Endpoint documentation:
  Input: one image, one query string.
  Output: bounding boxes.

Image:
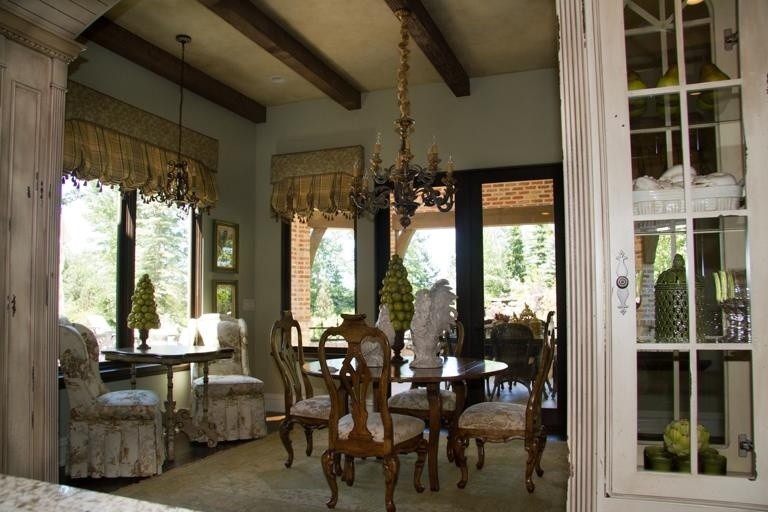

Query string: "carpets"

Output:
[111,420,569,510]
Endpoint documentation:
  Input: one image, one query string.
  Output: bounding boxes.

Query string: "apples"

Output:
[379,254,415,330]
[128,274,161,329]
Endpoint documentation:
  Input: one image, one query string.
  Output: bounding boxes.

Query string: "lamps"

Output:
[348,8,458,226]
[139,35,199,209]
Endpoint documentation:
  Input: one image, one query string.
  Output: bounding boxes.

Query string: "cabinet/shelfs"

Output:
[600,0,768,512]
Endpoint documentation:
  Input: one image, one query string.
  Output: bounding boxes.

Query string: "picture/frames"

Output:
[213,279,238,319]
[213,219,239,274]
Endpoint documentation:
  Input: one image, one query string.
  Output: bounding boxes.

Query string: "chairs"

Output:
[57,316,164,478]
[453,311,555,491]
[387,320,465,460]
[269,310,366,469]
[489,323,547,401]
[532,310,555,392]
[188,313,267,442]
[318,314,428,512]
